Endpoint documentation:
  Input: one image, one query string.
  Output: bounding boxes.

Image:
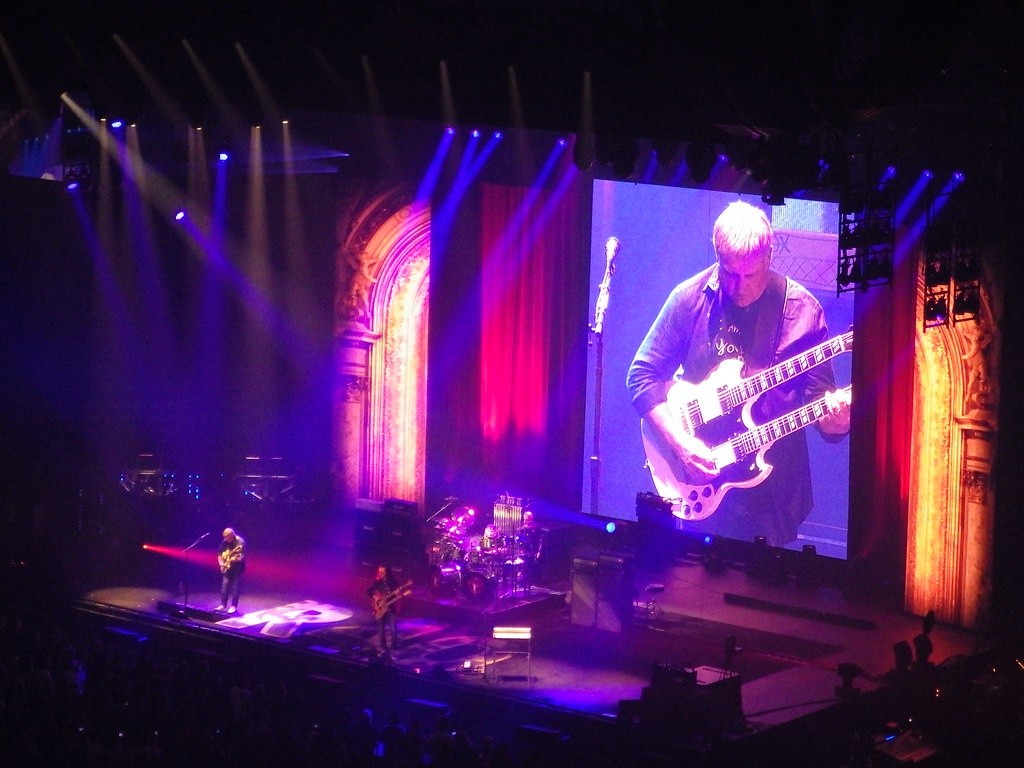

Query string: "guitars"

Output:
[641,325,853,522]
[373,579,413,620]
[220,545,242,573]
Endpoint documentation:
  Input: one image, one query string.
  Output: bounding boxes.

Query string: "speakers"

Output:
[572,551,635,634]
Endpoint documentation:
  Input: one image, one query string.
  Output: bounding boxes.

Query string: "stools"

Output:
[644,583,665,616]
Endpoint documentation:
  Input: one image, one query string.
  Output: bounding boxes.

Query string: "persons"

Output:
[623,202,848,546]
[517,511,541,582]
[374,563,400,664]
[217,528,248,610]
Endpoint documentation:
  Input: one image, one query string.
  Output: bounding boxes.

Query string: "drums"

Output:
[460,567,496,601]
[429,562,461,598]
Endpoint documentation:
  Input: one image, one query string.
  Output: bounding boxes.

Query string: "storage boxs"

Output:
[356,498,385,513]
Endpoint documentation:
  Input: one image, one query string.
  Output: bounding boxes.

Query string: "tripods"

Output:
[420,505,449,597]
[501,508,523,601]
[167,541,200,620]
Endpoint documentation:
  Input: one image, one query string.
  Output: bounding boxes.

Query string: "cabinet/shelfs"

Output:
[688,665,743,713]
[382,498,418,552]
[491,626,532,690]
[354,508,385,554]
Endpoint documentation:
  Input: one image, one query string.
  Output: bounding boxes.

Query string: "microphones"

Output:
[593,236,621,334]
[201,532,210,540]
